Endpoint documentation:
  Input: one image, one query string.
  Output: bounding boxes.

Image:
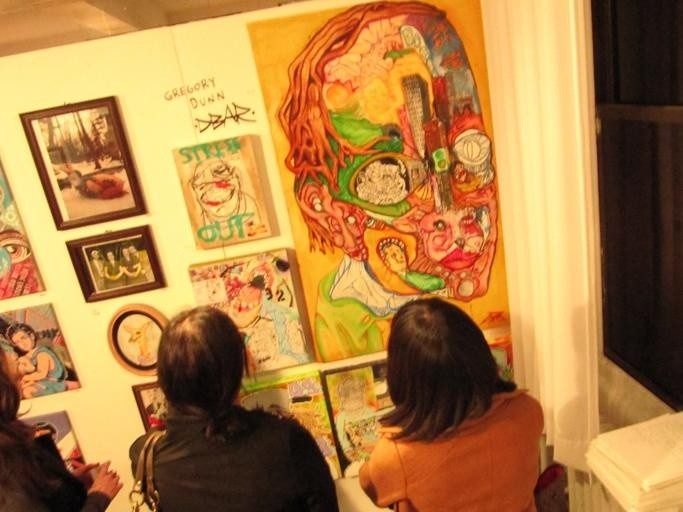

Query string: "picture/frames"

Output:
[66,225,167,304]
[107,303,172,376]
[132,381,169,436]
[18,95,147,231]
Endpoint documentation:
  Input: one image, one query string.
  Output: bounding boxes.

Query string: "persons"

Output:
[5,323,68,399]
[1,353,123,512]
[359,299,544,512]
[128,306,337,511]
